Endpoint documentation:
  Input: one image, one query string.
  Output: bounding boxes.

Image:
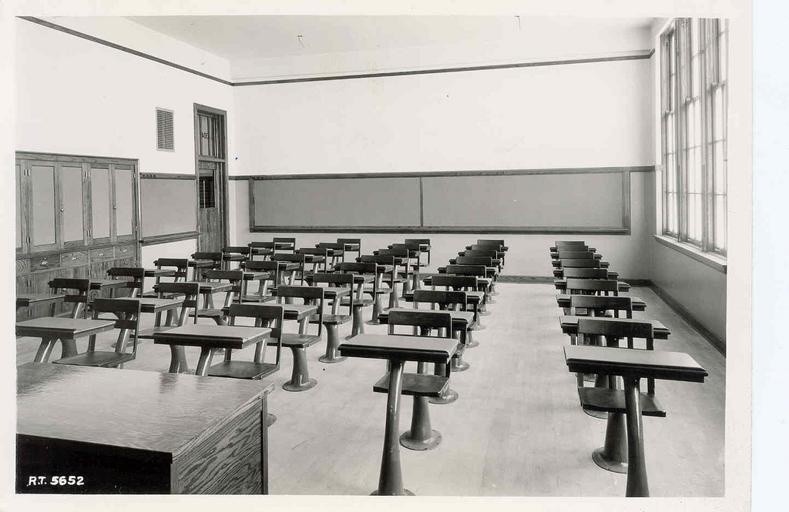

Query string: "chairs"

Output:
[16,238,708,497]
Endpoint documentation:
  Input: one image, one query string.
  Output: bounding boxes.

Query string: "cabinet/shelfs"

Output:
[15,151,142,335]
